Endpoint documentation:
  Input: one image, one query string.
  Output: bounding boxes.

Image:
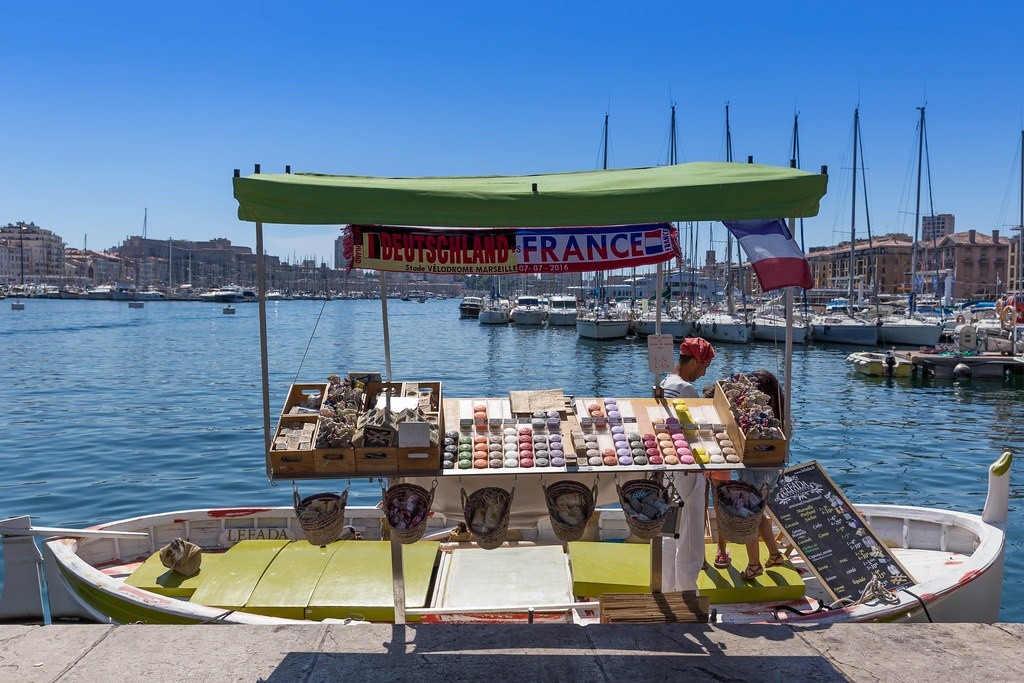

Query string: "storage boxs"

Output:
[713,377,787,463]
[269,381,442,475]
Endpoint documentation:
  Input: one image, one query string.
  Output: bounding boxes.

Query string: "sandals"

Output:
[744,562,763,582]
[714,550,730,566]
[765,552,784,566]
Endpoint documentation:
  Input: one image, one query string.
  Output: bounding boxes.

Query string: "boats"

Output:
[847,352,913,377]
[42,450,1016,625]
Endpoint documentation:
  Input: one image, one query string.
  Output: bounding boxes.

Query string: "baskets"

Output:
[294,491,348,546]
[382,483,433,544]
[616,479,674,539]
[711,480,770,544]
[461,487,515,550]
[542,480,598,542]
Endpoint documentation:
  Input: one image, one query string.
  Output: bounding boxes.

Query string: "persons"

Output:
[700,384,730,569]
[740,370,785,580]
[661,337,714,600]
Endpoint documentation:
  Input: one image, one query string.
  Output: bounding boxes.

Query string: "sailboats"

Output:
[0,254,456,304]
[457,99,1024,353]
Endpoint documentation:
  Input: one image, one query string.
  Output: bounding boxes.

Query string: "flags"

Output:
[722,219,814,291]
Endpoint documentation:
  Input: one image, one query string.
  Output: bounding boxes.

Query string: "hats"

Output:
[679,337,715,362]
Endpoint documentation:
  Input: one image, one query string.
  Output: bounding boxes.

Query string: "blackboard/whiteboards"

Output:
[763,459,919,608]
